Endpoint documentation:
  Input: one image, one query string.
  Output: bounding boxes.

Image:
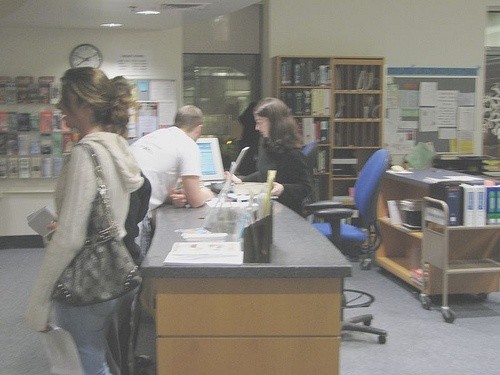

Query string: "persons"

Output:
[226,97,314,218]
[131,104,213,365]
[26,68,144,375]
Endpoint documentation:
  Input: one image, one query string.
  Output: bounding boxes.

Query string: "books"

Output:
[281,61,330,200]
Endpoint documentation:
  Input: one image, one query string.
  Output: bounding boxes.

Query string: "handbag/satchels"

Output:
[52,142,142,305]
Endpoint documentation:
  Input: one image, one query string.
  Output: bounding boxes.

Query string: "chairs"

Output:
[305,147,394,343]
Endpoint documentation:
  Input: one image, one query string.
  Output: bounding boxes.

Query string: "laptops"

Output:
[193,137,225,187]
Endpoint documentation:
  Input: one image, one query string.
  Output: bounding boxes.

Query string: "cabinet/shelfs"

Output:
[375,164,488,295]
[422,197,500,304]
[331,55,387,202]
[273,53,332,202]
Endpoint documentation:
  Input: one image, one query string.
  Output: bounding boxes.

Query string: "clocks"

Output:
[68,43,105,71]
